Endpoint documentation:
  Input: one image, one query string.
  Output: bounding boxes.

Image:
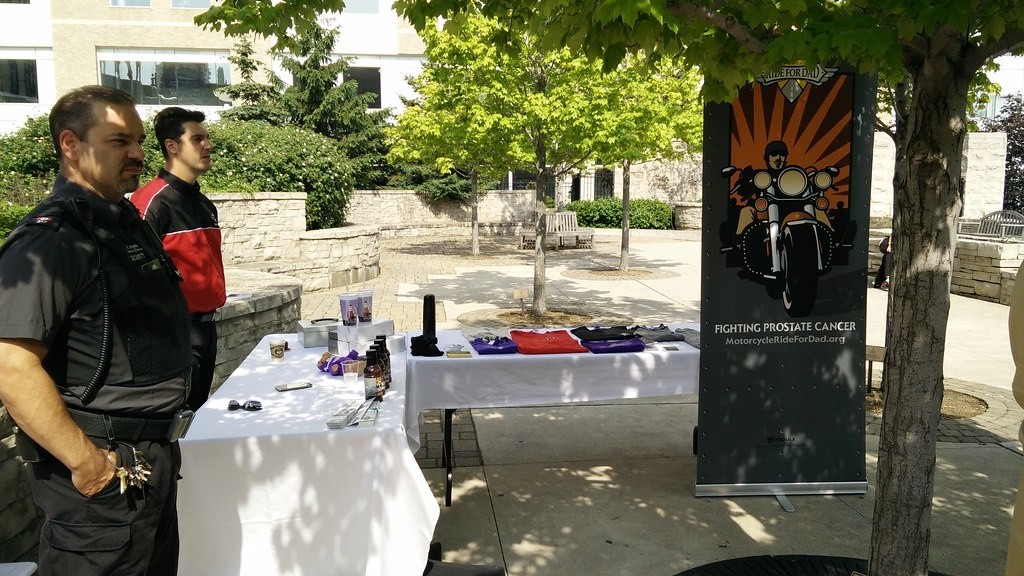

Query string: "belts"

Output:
[190,312,215,323]
[68,410,191,443]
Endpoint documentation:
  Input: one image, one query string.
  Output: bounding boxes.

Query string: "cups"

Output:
[339,293,357,325]
[269,341,286,363]
[355,291,372,322]
[341,360,359,385]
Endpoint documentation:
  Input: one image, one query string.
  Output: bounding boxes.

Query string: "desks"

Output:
[177,333,440,576]
[405,325,700,507]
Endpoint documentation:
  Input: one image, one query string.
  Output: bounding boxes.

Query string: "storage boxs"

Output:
[349,335,407,356]
[338,320,395,343]
[297,319,342,348]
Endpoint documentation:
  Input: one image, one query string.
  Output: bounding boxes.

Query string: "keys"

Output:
[116,450,151,494]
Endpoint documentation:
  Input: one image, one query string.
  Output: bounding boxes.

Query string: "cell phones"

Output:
[275,382,312,392]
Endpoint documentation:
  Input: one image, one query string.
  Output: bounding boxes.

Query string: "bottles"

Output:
[365,334,392,402]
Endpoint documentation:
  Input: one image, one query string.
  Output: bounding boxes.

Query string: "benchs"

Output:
[519,213,559,251]
[958,210,1024,244]
[557,212,595,249]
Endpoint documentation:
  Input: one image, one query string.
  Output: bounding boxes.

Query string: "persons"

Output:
[0,83,196,576]
[128,106,227,413]
